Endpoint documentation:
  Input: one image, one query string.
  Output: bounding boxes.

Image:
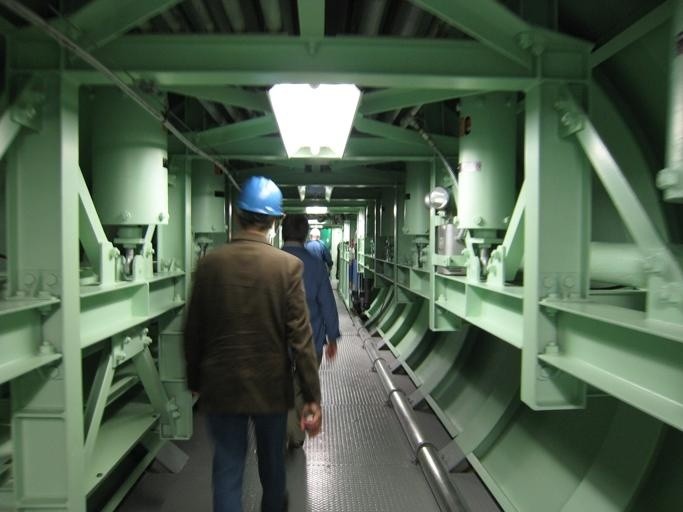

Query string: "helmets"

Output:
[236,176,284,218]
[309,228,320,237]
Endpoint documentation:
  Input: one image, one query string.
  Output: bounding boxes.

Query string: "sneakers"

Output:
[281,437,304,454]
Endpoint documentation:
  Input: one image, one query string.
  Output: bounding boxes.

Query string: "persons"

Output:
[179,173,323,511]
[279,212,342,448]
[306,228,333,277]
